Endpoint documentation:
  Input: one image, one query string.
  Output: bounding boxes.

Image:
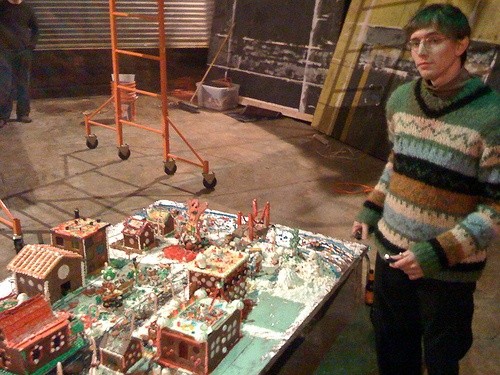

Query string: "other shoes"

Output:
[0,116,7,127]
[17,115,33,122]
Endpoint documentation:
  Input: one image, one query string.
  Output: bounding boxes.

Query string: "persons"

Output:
[351,4,500,375]
[0,0,39,129]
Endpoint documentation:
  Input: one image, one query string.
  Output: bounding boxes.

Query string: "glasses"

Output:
[407,35,450,47]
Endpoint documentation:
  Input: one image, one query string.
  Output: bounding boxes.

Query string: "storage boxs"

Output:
[196,80,241,111]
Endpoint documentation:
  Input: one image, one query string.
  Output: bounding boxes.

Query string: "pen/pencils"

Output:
[385,254,394,263]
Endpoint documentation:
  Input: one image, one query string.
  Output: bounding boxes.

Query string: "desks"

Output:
[0,199,368,375]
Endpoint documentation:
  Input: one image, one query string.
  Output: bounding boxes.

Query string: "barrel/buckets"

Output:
[110,73,138,122]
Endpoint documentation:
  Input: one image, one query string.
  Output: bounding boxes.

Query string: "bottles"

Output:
[364,269,375,305]
